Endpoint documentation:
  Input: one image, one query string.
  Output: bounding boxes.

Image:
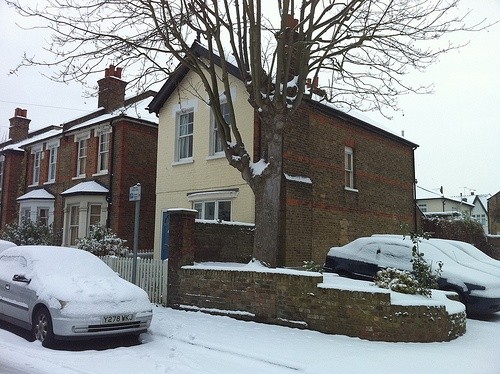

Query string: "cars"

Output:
[326,236,499,318]
[0,246,153,348]
[1,239,15,253]
